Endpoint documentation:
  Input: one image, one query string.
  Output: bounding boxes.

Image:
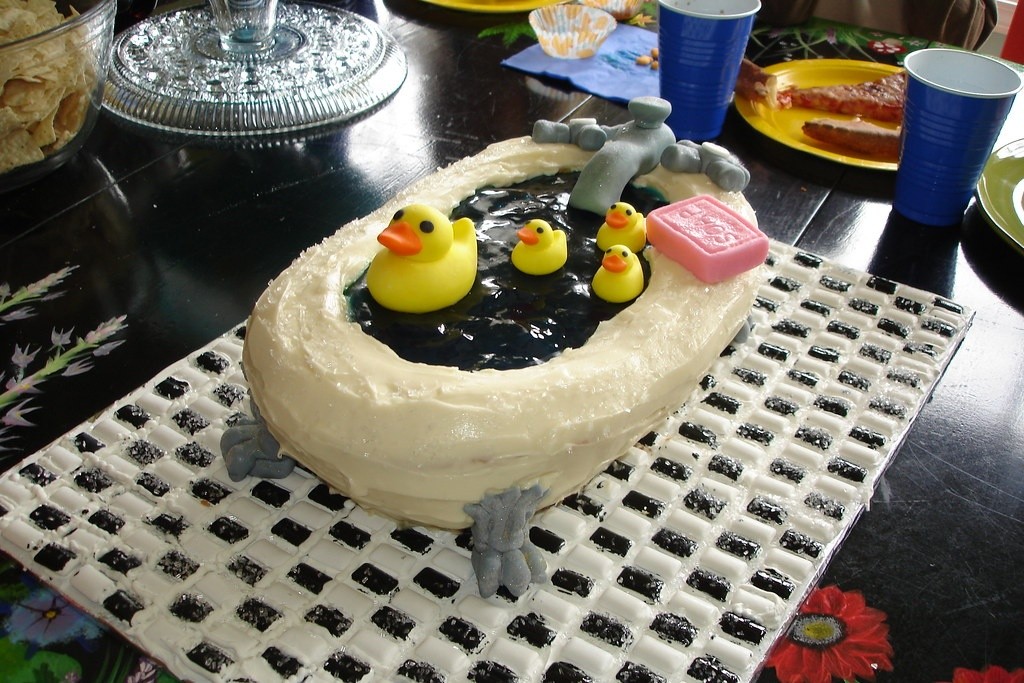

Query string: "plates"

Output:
[422,0,573,13]
[734,58,906,172]
[975,138,1024,255]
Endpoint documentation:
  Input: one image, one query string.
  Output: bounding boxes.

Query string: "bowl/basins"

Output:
[0,0,118,193]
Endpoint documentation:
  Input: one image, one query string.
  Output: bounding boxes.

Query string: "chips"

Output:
[0,0,104,172]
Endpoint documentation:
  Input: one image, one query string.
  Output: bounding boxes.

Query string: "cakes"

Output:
[216,95,769,597]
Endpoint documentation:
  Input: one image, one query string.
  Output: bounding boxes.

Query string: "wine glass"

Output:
[100,0,408,150]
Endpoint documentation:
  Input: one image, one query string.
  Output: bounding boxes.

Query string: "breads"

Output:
[780,70,906,120]
[801,117,900,156]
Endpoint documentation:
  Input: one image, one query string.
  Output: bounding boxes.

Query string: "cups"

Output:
[891,48,1024,226]
[658,0,761,142]
